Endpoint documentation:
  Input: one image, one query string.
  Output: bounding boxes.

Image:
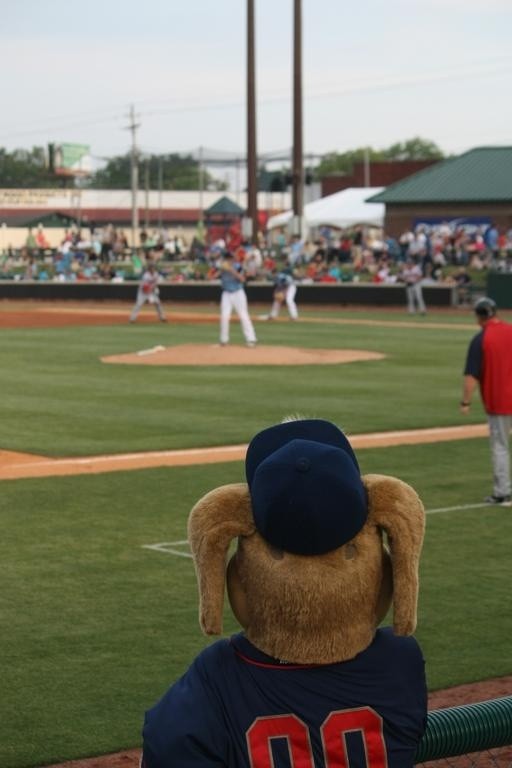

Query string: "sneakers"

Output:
[484,495,511,507]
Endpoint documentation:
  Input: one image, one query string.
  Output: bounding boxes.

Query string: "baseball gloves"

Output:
[275,291,285,302]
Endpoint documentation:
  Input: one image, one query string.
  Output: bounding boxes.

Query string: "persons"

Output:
[401,259,427,316]
[266,270,298,320]
[460,297,512,507]
[213,252,257,349]
[129,264,168,324]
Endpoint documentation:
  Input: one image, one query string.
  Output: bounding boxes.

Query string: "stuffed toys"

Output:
[140,414,428,768]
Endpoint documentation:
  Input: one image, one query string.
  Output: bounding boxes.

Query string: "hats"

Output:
[475,297,496,319]
[246,420,368,556]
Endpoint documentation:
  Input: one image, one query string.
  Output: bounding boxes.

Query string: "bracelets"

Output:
[461,401,471,406]
